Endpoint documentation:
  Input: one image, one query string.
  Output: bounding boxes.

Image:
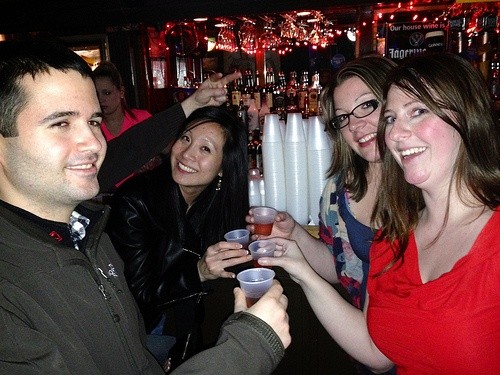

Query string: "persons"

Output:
[246,53,398,310]
[0,40,291,374]
[92,61,153,142]
[97,71,242,197]
[110,106,254,341]
[257,46,500,374]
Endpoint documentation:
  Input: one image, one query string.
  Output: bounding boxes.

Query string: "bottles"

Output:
[184,68,323,174]
[479,32,500,101]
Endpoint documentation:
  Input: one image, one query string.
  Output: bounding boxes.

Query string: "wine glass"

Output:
[214,10,328,54]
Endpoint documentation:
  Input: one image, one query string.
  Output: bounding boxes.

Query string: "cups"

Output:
[248,240,276,269]
[224,229,250,251]
[248,113,335,225]
[252,206,277,236]
[237,267,275,308]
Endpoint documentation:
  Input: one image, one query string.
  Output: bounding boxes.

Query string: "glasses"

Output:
[327,99,380,130]
